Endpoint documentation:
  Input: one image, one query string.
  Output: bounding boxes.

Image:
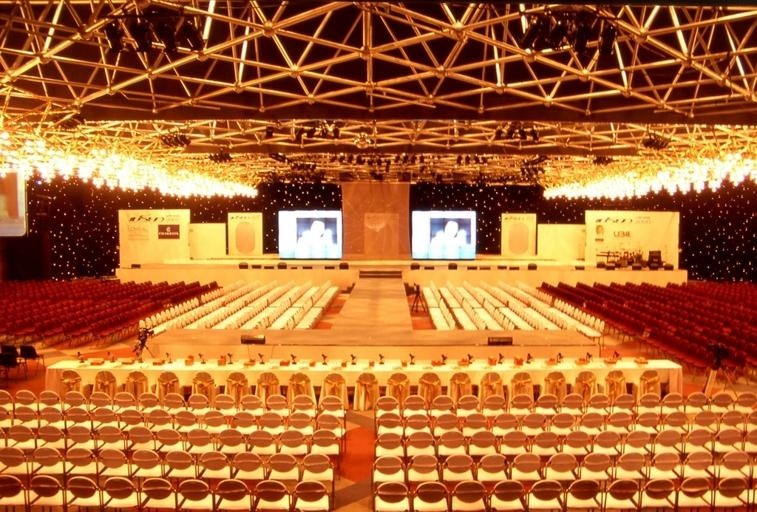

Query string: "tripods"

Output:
[132,343,155,358]
[412,295,426,313]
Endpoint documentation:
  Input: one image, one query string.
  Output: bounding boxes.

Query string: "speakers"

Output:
[487,336,513,345]
[649,250,662,268]
[240,334,265,344]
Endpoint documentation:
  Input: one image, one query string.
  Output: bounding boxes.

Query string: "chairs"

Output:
[0,279,757,512]
